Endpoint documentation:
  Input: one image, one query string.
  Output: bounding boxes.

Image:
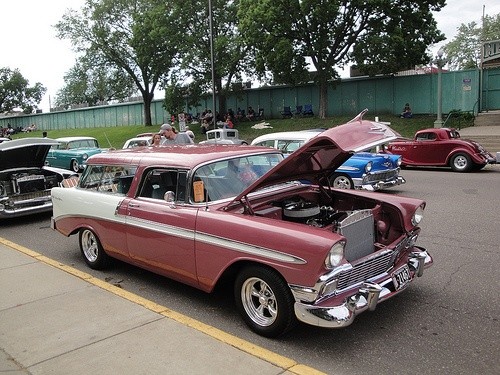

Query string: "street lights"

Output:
[433,49,447,128]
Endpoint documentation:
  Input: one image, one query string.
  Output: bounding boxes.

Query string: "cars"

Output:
[197,129,250,147]
[0,139,80,222]
[378,127,497,172]
[45,136,116,172]
[120,131,196,177]
[50,108,435,337]
[243,128,406,192]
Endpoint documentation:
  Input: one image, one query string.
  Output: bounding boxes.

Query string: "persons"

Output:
[22,122,36,133]
[151,133,162,146]
[201,119,209,133]
[234,107,243,121]
[4,127,17,133]
[400,102,411,118]
[185,107,236,128]
[158,123,195,143]
[178,113,186,131]
[166,111,175,125]
[245,105,256,121]
[184,126,195,141]
[43,131,48,138]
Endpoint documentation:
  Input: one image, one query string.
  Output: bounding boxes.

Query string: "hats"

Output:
[158,124,171,134]
